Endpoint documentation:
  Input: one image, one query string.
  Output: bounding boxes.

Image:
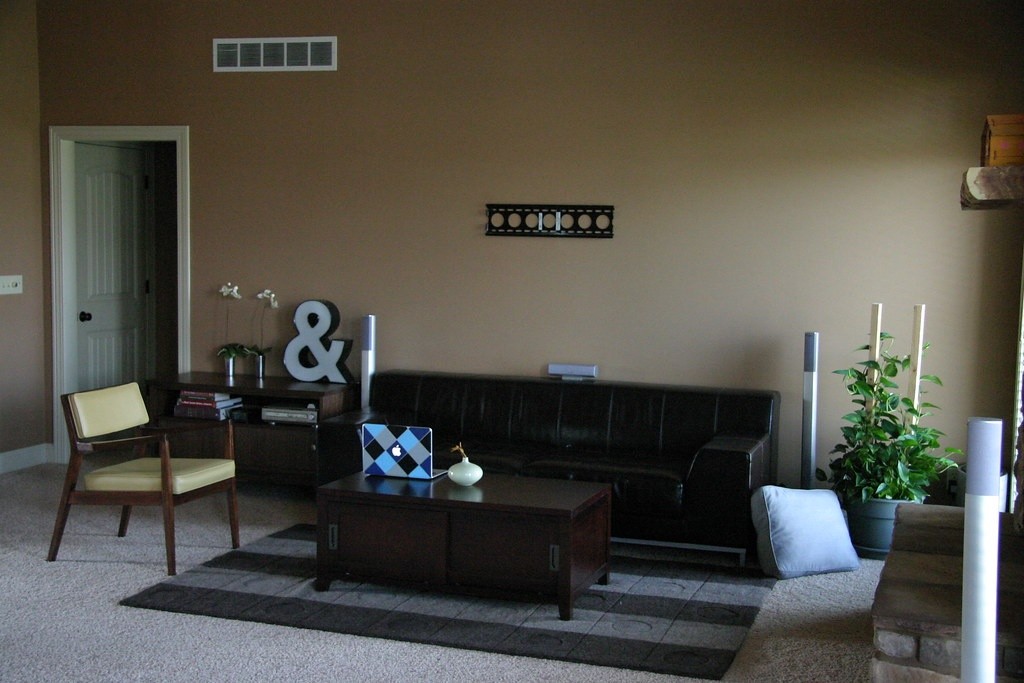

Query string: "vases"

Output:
[223,357,234,376]
[253,355,265,378]
[448,457,483,486]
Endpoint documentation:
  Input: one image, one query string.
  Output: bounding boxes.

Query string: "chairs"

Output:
[47,381,240,575]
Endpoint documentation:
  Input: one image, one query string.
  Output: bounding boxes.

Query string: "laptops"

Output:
[362,423,448,479]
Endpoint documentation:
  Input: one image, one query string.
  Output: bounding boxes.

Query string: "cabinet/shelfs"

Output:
[146,372,360,492]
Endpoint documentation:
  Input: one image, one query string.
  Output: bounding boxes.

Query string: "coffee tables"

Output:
[316,471,613,621]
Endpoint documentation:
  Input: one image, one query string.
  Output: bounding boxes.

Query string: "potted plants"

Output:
[815,332,964,561]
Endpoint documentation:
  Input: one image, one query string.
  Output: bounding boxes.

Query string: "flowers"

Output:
[216,282,246,359]
[249,289,279,356]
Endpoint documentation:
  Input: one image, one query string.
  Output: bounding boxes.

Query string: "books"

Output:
[174,391,242,421]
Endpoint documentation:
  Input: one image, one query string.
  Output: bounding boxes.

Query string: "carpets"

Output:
[116,524,778,683]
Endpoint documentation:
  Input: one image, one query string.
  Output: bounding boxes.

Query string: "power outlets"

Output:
[0,274,23,294]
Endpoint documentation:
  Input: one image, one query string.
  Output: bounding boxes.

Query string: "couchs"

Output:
[317,368,780,565]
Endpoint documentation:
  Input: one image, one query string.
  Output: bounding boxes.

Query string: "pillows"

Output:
[751,486,860,580]
